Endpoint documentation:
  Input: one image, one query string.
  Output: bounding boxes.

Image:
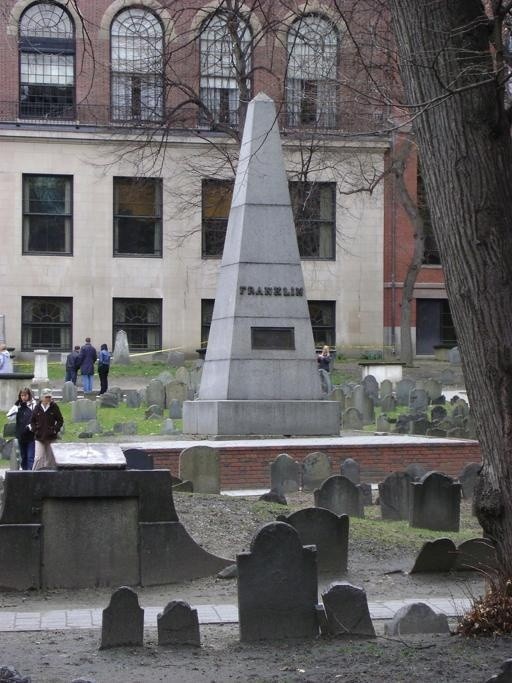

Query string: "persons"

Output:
[29,388,65,471]
[315,344,334,393]
[0,342,14,373]
[6,385,39,470]
[64,344,81,386]
[76,337,97,393]
[95,343,111,395]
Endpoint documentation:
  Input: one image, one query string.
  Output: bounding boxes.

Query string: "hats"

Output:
[40,387,53,398]
